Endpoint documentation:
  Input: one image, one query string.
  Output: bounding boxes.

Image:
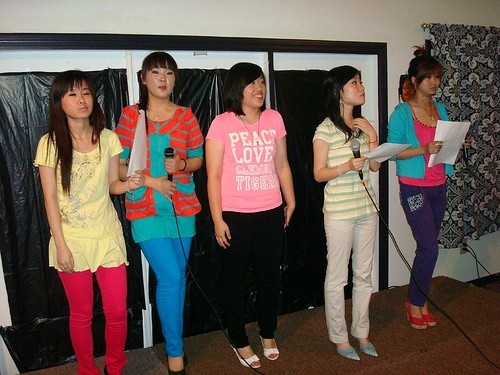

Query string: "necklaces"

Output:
[237,110,260,145]
[413,96,435,121]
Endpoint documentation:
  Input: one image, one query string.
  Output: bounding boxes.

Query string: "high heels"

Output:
[421,312,438,326]
[405,300,428,329]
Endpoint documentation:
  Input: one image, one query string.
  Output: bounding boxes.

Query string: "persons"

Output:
[385,44,454,329]
[312,65,381,361]
[34,68,146,375]
[114,51,204,375]
[206,62,296,369]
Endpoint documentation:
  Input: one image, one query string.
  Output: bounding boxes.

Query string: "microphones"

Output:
[165,148,174,183]
[349,139,363,180]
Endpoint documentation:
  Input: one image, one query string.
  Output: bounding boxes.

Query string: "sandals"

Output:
[258,334,280,360]
[230,343,261,369]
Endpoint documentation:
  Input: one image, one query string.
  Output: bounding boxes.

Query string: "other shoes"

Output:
[166,349,187,375]
[337,346,361,361]
[360,342,379,357]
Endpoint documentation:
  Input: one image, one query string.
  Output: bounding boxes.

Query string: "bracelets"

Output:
[126,181,136,194]
[337,164,345,176]
[368,137,377,145]
[181,158,187,171]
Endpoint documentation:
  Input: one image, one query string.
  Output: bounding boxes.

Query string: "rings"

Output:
[168,191,172,193]
[64,267,68,269]
